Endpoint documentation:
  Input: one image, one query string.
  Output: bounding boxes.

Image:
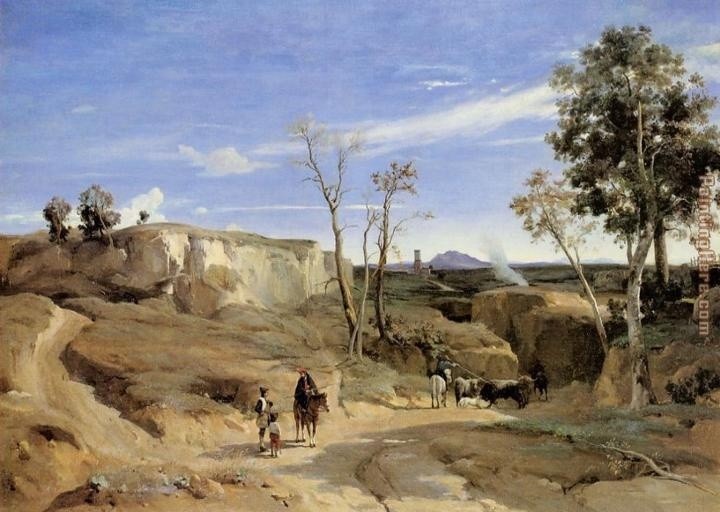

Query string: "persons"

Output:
[253,384,274,452]
[268,410,282,459]
[293,367,319,418]
[435,354,461,392]
[529,357,544,379]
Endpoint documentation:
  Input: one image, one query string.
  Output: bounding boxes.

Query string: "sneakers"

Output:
[259,446,269,452]
[269,454,281,458]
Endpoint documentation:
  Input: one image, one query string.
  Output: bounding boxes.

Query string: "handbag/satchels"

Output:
[256,412,269,429]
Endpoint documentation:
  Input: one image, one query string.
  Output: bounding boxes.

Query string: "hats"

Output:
[296,368,308,373]
[260,386,268,393]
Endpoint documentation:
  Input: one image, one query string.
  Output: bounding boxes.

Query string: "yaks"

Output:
[454,375,538,410]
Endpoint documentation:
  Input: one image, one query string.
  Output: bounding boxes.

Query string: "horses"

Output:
[292,392,330,448]
[429,367,451,409]
[527,365,549,401]
